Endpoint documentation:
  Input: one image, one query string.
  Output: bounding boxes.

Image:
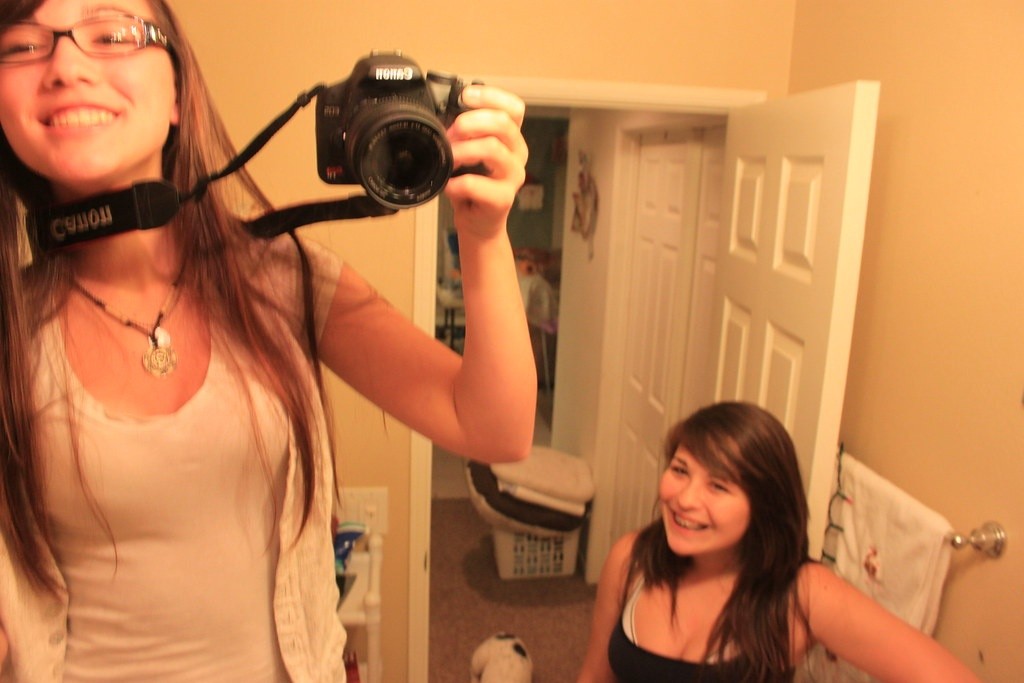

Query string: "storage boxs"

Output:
[491,522,580,580]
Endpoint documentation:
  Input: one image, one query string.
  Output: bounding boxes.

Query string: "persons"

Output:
[0,0,537,683]
[577,402,981,683]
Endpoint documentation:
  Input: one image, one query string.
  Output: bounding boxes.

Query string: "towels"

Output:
[487,442,595,517]
[823,451,951,660]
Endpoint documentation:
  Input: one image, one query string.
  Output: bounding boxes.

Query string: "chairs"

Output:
[436,227,466,353]
[330,485,389,683]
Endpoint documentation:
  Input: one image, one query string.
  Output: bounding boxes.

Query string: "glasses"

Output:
[0,15,174,64]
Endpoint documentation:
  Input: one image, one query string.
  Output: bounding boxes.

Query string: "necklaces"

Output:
[70,253,189,377]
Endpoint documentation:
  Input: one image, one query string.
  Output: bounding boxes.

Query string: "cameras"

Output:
[314,54,485,211]
